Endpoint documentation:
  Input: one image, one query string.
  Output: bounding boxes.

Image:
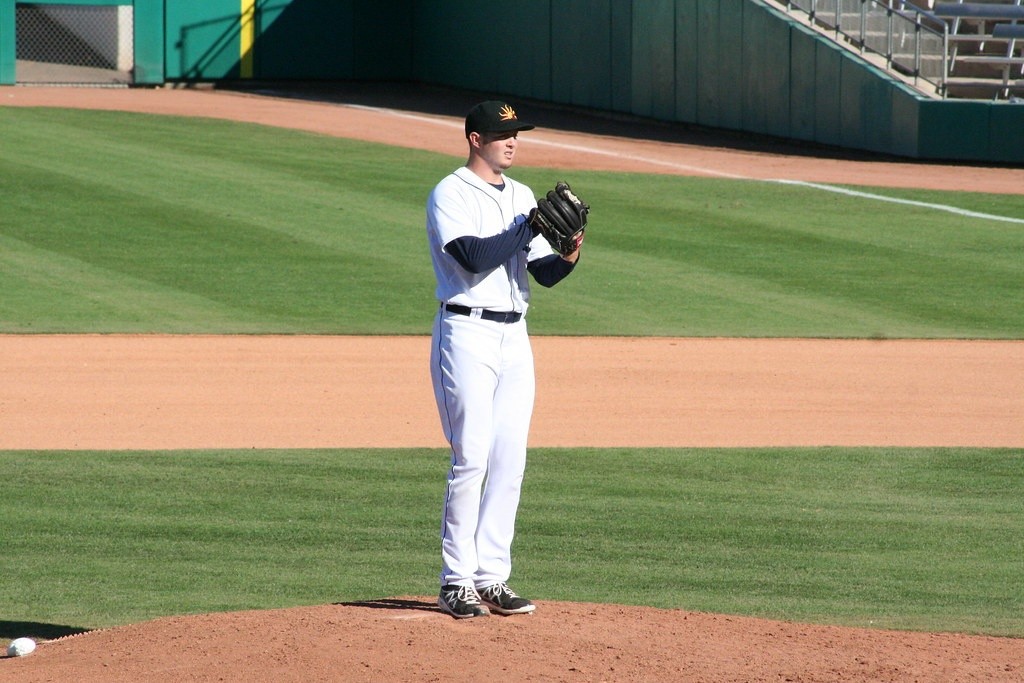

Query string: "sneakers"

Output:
[477,582,536,614]
[438,584,489,617]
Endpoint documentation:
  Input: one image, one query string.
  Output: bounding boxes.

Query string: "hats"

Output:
[465,101,535,132]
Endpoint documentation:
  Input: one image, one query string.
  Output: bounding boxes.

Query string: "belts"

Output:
[441,301,521,324]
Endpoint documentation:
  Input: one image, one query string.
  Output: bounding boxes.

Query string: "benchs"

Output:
[917,0,1024,91]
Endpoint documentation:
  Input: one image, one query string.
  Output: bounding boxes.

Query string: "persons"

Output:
[426,102,581,619]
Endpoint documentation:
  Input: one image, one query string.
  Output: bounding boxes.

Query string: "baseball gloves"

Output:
[529,178,591,257]
